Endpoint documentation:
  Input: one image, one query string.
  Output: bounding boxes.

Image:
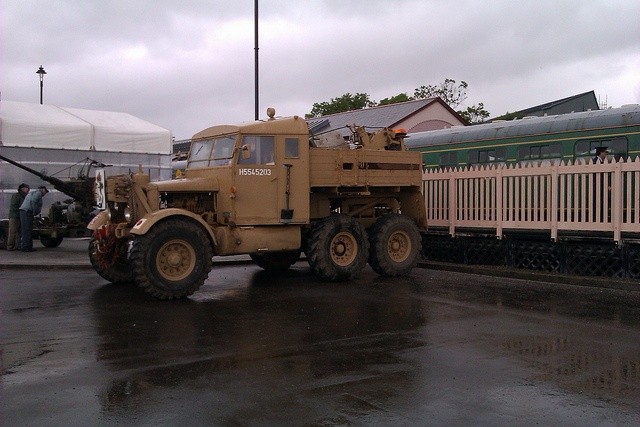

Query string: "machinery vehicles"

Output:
[0,155,113,248]
[89,108,427,299]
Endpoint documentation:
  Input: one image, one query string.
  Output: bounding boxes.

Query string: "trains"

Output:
[403,105,640,211]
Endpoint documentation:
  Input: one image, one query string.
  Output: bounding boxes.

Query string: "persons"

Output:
[6,182,30,250]
[592,144,608,221]
[17,184,50,250]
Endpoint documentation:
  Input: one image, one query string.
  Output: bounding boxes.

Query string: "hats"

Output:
[38,186,49,193]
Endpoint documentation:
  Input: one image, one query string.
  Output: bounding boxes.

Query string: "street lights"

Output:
[36,64,47,104]
